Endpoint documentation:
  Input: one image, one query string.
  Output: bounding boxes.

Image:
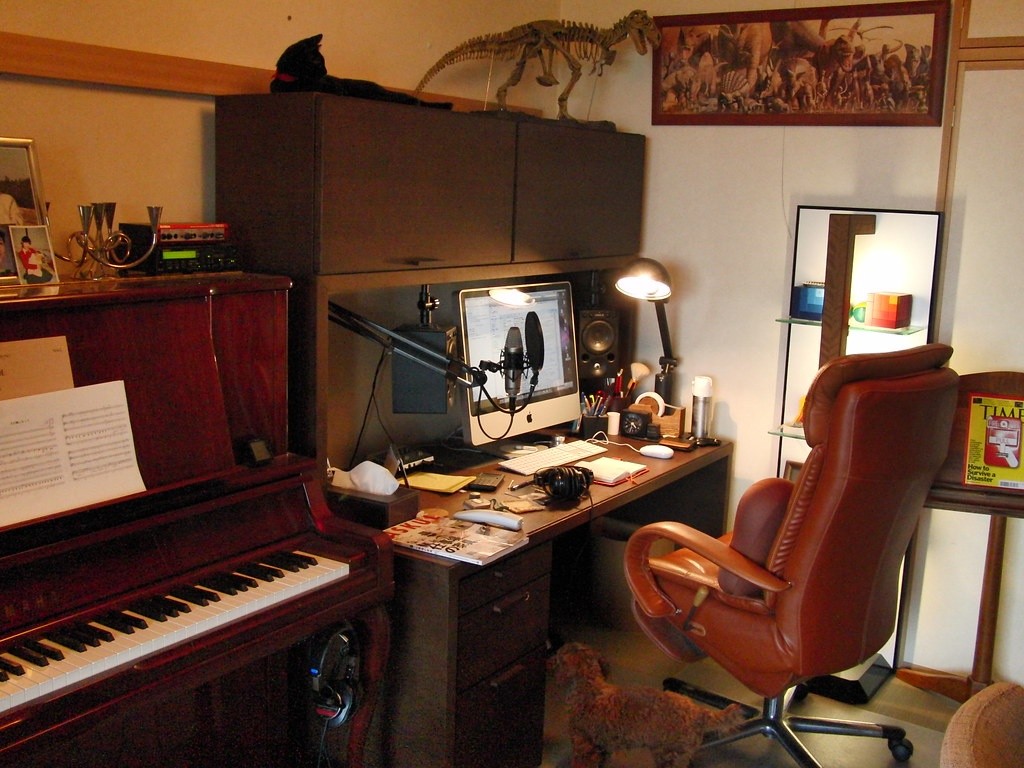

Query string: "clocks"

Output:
[620,410,652,438]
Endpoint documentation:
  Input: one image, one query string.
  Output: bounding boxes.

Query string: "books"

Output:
[574,457,649,486]
[383,515,530,566]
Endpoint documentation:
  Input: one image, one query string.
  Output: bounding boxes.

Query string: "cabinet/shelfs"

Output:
[214,91,647,280]
[767,313,926,440]
[386,428,734,768]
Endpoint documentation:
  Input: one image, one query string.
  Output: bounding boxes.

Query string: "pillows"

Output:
[717,479,796,597]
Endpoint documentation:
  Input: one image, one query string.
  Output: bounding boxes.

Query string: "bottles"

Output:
[691,376,713,439]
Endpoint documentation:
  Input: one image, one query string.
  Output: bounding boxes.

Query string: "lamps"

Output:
[613,258,679,415]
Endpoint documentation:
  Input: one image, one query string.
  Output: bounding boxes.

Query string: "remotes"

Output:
[454,509,523,531]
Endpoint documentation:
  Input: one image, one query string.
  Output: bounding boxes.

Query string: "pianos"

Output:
[0,268,397,768]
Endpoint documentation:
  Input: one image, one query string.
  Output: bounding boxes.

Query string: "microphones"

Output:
[504,327,524,415]
[525,310,545,395]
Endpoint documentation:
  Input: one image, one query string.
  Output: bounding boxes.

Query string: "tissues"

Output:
[324,460,419,529]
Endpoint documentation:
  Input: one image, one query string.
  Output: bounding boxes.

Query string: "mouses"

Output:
[640,444,674,459]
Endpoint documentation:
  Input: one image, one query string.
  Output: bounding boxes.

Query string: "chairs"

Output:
[938,681,1024,768]
[622,342,961,768]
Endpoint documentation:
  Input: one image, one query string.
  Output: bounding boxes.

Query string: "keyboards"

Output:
[497,440,609,476]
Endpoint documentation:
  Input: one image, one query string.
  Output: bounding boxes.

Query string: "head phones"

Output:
[309,622,365,730]
[534,466,594,505]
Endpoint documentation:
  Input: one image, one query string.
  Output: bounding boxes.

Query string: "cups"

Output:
[610,398,632,432]
[583,414,608,440]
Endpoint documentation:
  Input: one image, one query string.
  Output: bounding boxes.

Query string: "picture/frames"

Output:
[651,0,952,127]
[8,225,61,285]
[0,137,52,286]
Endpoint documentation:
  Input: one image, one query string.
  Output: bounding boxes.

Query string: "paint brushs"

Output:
[625,363,650,397]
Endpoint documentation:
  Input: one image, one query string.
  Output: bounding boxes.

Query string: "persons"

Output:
[0,230,15,276]
[18,236,53,284]
[0,193,24,225]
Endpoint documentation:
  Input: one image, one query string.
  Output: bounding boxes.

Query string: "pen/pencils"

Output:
[580,390,612,416]
[507,479,514,489]
[512,480,532,491]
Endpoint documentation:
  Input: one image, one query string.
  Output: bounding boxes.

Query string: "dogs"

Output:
[542,641,745,768]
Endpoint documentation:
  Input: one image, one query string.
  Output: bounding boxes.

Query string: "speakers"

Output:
[574,308,619,380]
[392,323,457,415]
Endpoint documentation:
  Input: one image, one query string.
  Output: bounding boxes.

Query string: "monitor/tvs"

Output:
[453,280,581,460]
